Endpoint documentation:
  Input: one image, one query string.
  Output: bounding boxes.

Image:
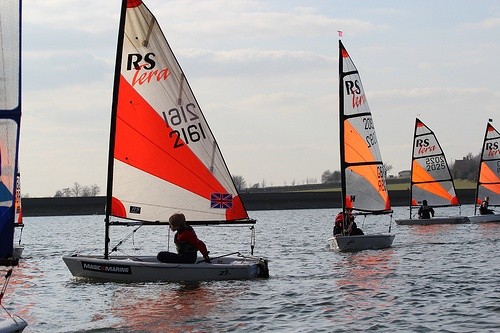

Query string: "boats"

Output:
[0,268,27,333]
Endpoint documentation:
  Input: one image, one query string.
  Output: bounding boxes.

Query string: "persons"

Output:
[479,196,495,215]
[418,200,435,219]
[333,207,364,236]
[156,214,212,264]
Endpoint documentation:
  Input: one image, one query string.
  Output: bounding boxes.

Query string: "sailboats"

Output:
[328,30,395,250]
[467,119,500,222]
[62,0,269,283]
[394,117,466,225]
[1,1,21,267]
[16,169,25,260]
